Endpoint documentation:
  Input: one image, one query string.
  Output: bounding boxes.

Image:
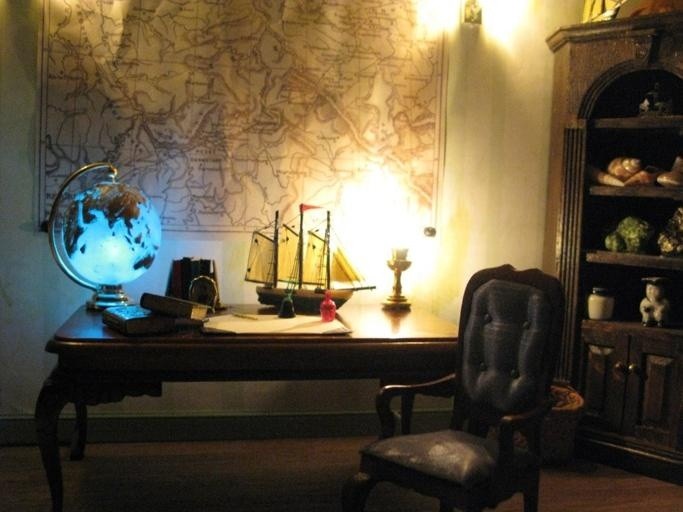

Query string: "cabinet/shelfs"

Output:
[545,322,682,487]
[546,0,683,329]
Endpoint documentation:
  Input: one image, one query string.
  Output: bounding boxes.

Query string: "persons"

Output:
[640,277,670,330]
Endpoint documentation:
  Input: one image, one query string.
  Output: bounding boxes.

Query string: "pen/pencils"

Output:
[233,313,258,321]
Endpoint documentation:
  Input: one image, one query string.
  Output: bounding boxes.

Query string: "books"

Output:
[102,293,211,339]
[166,257,222,312]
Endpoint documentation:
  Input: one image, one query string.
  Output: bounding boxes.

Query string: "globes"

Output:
[47,161,162,311]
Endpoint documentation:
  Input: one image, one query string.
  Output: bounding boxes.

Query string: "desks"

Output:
[34,292,460,511]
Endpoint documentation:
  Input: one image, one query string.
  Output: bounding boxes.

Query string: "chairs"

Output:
[343,262,567,511]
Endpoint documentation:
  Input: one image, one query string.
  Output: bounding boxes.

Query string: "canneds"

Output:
[586,286,614,320]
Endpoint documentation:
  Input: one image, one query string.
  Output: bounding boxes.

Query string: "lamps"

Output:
[381,249,413,314]
[460,0,483,29]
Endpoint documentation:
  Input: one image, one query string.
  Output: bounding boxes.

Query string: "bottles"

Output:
[319,290,336,322]
